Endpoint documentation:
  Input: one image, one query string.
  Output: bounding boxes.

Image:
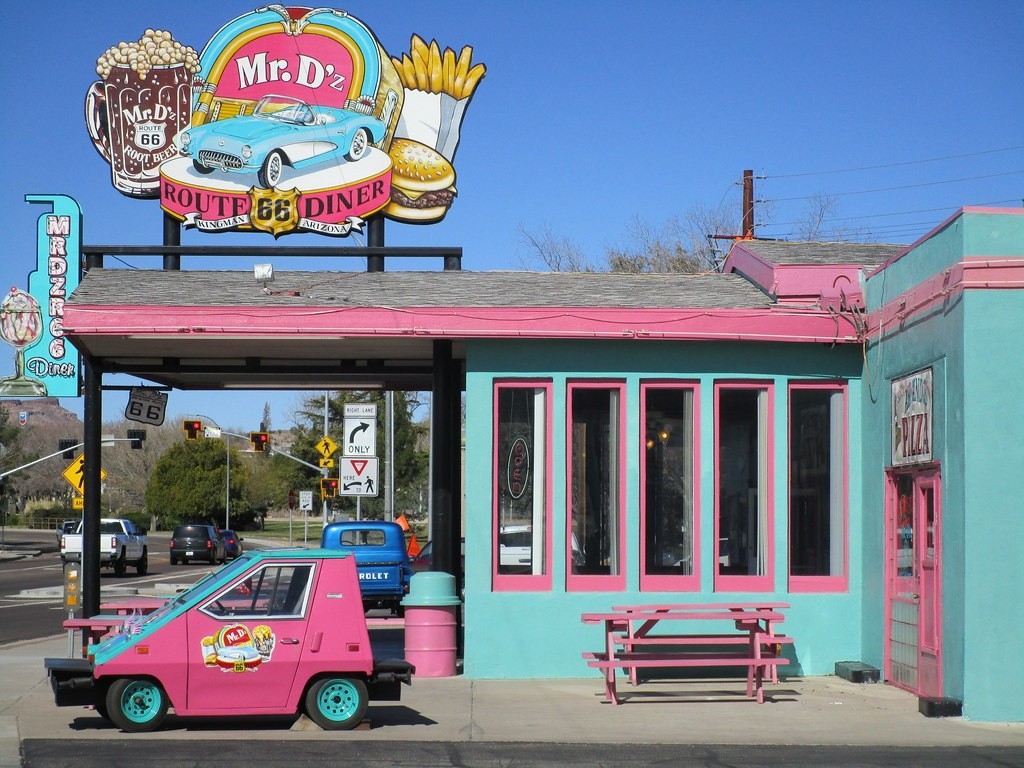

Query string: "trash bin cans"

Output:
[400,570,462,680]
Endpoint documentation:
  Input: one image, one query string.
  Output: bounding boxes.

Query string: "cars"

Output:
[56,520,78,548]
[218,529,244,560]
[43,545,415,730]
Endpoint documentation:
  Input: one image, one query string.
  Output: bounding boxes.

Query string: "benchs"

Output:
[581,634,794,666]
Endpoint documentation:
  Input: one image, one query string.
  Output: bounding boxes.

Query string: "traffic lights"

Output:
[183,420,202,441]
[250,431,270,452]
[320,478,338,498]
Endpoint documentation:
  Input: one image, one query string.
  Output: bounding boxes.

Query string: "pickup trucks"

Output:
[320,521,416,618]
[60,519,149,578]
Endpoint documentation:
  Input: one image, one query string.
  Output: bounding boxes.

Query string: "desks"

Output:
[581,613,787,705]
[612,602,791,686]
[62,599,274,638]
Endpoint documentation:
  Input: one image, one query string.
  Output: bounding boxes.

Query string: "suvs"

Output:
[170,524,228,566]
